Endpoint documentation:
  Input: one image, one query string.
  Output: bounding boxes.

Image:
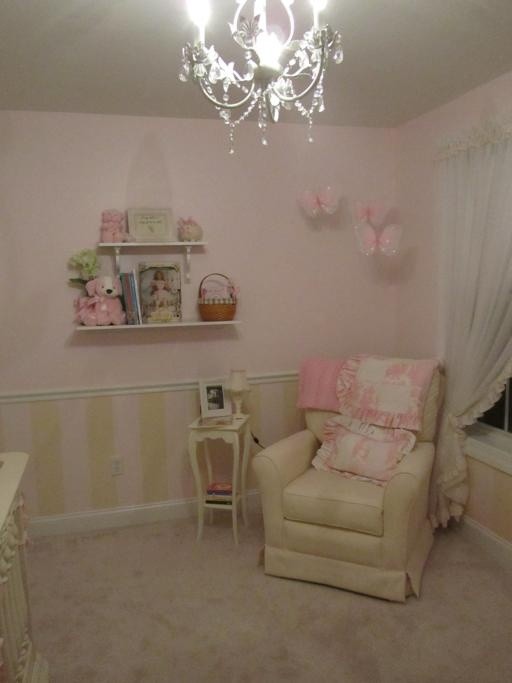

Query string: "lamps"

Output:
[225,371,251,419]
[178,0,346,158]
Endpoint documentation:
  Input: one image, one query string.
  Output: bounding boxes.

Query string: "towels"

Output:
[294,355,346,414]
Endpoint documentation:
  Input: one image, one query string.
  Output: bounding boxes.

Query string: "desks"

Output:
[189,413,252,548]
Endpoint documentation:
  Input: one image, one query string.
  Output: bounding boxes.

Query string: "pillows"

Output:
[310,416,419,485]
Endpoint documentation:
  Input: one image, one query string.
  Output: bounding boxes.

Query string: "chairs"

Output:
[255,354,447,603]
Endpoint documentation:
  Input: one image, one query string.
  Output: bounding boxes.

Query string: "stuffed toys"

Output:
[176,216,203,241]
[77,274,128,327]
[100,207,127,243]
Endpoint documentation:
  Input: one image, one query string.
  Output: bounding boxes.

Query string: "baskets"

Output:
[198,273,237,321]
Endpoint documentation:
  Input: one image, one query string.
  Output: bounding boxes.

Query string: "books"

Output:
[119,268,144,326]
[206,482,239,506]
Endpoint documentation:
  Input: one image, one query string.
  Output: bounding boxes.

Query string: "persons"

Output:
[144,269,175,313]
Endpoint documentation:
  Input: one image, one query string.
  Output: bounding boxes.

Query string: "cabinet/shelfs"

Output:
[71,242,243,332]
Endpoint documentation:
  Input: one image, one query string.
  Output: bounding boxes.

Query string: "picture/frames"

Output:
[199,376,231,420]
[127,208,174,240]
